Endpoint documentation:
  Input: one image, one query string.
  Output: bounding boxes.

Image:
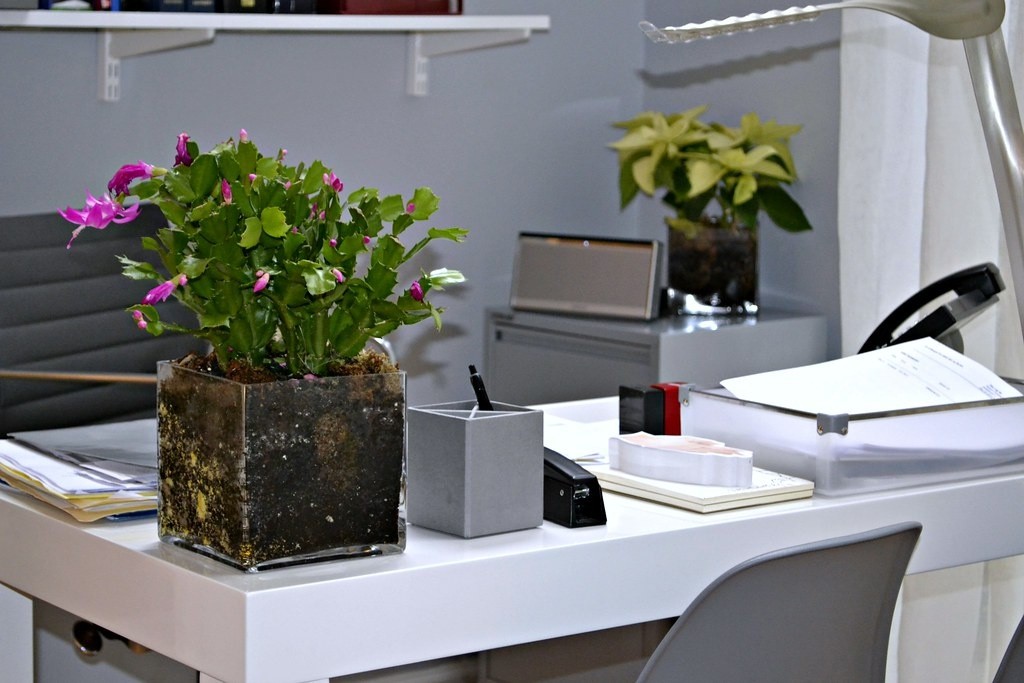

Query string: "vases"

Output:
[155,359,409,575]
[667,223,759,316]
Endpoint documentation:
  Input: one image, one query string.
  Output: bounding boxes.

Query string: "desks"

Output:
[0,397,1024,683]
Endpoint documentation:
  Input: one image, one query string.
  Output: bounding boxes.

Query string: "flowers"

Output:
[603,103,814,240]
[54,124,470,379]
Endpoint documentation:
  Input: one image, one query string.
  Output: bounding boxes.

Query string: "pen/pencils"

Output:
[466,364,494,410]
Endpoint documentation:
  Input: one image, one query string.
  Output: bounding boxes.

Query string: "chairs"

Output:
[635,521,923,683]
[0,203,213,438]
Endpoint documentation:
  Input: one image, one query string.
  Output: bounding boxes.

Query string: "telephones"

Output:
[855,261,1007,357]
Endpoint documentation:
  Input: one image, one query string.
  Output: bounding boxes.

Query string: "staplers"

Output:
[542,443,608,530]
